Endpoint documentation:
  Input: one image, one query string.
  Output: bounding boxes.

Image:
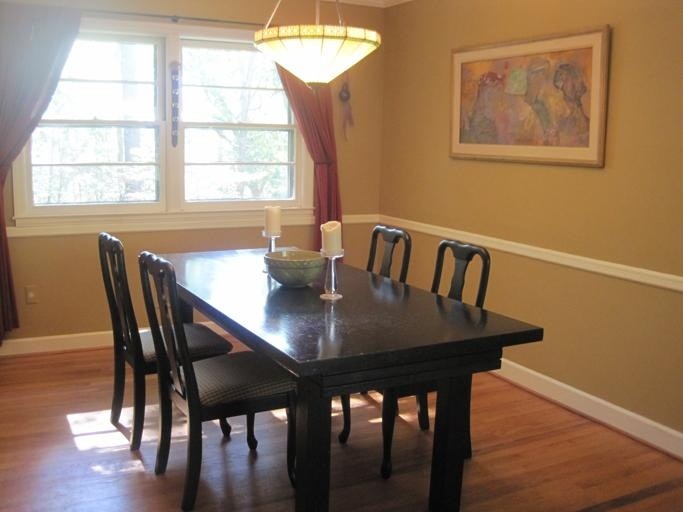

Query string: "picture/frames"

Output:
[448,23,612,168]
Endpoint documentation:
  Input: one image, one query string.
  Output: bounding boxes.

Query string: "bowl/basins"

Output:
[264,249,327,289]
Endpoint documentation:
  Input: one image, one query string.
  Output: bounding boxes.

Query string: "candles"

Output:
[321,219,343,256]
[263,204,282,234]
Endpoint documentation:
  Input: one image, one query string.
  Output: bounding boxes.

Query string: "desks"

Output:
[157,245,544,512]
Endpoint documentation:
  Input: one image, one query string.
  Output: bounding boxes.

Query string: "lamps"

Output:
[254,0,379,97]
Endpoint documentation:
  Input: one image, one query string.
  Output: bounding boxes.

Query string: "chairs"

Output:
[366,225,413,282]
[139,251,298,512]
[339,240,491,480]
[97,232,232,452]
